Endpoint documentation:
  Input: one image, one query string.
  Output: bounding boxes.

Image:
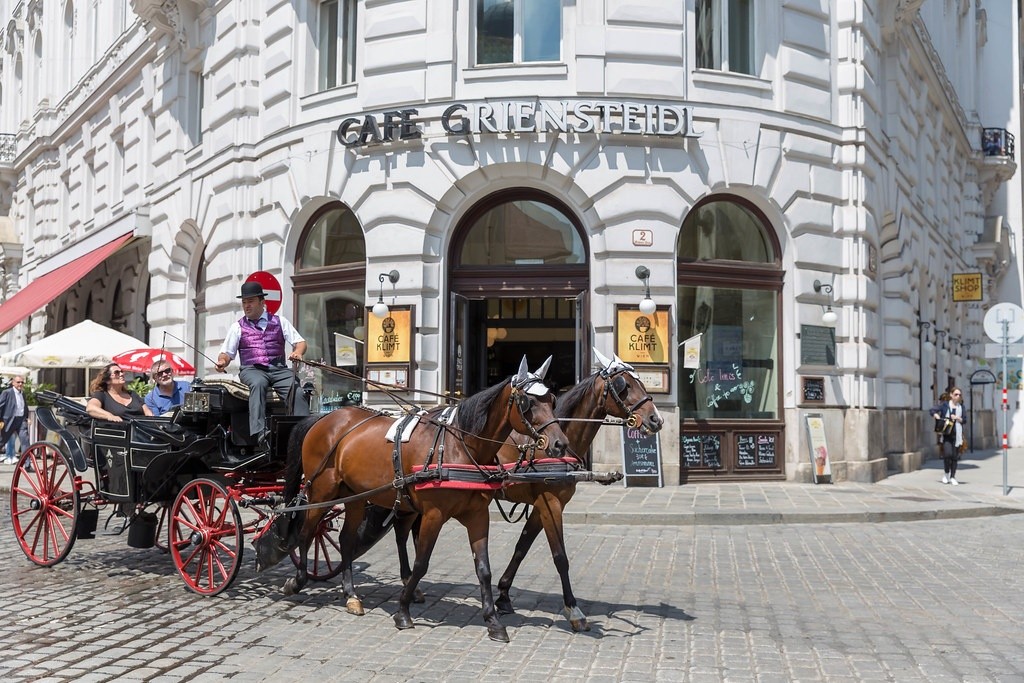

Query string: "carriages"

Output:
[9,345,666,643]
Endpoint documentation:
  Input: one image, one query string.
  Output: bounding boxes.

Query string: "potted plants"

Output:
[22,377,57,457]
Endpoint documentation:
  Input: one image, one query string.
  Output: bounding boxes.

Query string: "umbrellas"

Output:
[112,348,196,387]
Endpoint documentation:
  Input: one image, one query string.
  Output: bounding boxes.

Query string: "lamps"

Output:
[354,304,364,340]
[635,266,657,315]
[917,321,973,368]
[813,280,837,322]
[372,270,400,317]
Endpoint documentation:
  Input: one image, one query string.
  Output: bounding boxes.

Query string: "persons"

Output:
[86,362,154,422]
[0,377,37,473]
[144,360,191,416]
[215,283,310,445]
[4,431,20,465]
[930,388,967,486]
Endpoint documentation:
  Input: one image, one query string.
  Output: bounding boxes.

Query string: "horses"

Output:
[284,353,570,643]
[360,347,664,631]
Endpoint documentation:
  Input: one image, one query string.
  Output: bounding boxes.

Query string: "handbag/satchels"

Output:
[933,402,954,435]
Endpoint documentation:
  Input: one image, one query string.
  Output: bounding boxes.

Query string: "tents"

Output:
[2,320,152,397]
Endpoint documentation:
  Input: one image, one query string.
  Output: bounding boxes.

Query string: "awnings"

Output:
[0,232,133,333]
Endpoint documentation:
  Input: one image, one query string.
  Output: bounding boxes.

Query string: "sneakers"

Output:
[943,473,949,483]
[4,459,12,464]
[950,478,959,486]
[12,457,20,464]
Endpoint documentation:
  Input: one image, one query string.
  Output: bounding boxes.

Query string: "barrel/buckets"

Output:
[77,501,99,540]
[128,507,158,548]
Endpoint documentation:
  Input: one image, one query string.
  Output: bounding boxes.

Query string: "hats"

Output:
[236,283,268,298]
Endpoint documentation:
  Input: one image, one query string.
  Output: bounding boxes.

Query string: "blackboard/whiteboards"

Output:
[702,435,721,468]
[758,435,776,466]
[737,435,755,466]
[681,435,701,468]
[620,424,662,477]
[800,376,825,404]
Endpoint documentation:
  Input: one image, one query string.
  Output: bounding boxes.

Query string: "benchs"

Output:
[194,383,286,416]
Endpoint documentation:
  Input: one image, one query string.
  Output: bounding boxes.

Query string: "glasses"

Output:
[153,368,170,378]
[109,369,124,377]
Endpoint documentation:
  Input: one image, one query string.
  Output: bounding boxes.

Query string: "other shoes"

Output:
[26,466,36,472]
[256,430,270,444]
